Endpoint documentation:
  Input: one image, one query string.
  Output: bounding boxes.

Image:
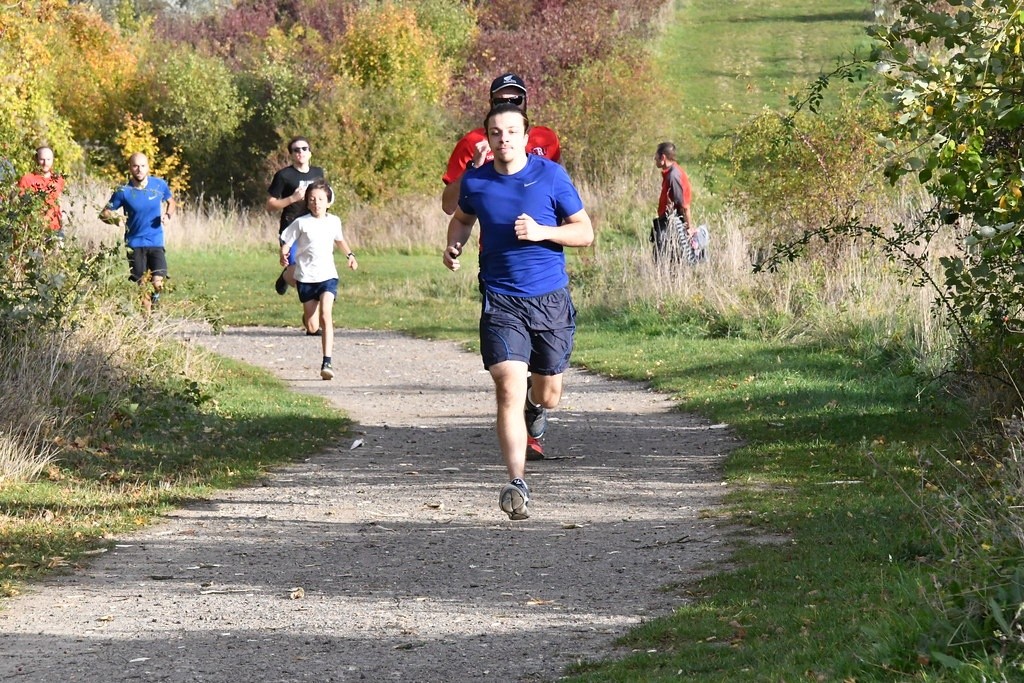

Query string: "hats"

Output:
[490,74,527,94]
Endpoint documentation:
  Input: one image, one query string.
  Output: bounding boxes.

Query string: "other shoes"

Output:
[306,329,322,335]
[151,291,159,306]
[276,266,289,294]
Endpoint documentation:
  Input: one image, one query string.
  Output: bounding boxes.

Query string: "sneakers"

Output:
[320,362,333,380]
[499,479,531,520]
[526,436,544,461]
[524,377,547,440]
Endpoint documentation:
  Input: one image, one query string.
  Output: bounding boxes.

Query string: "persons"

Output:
[98,151,174,331]
[441,105,595,518]
[453,74,565,459]
[651,143,691,266]
[278,180,357,380]
[15,147,67,266]
[265,136,335,335]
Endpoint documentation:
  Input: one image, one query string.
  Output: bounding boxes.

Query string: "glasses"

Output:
[294,147,308,152]
[491,95,525,105]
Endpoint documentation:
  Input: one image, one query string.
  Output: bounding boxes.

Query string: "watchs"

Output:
[165,212,171,220]
[347,253,355,258]
